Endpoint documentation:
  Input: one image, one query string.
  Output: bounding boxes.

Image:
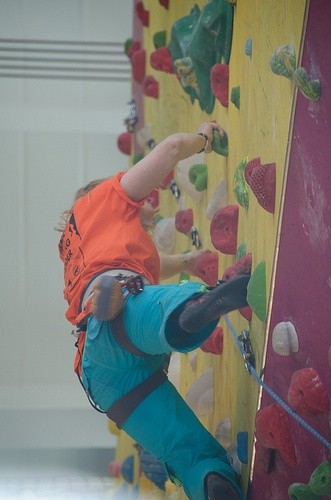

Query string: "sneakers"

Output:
[170,274,252,338]
[206,472,244,500]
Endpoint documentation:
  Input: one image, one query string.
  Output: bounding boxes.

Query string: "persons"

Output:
[57,120,251,500]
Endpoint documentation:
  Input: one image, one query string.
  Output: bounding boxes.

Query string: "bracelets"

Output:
[197,132,208,153]
